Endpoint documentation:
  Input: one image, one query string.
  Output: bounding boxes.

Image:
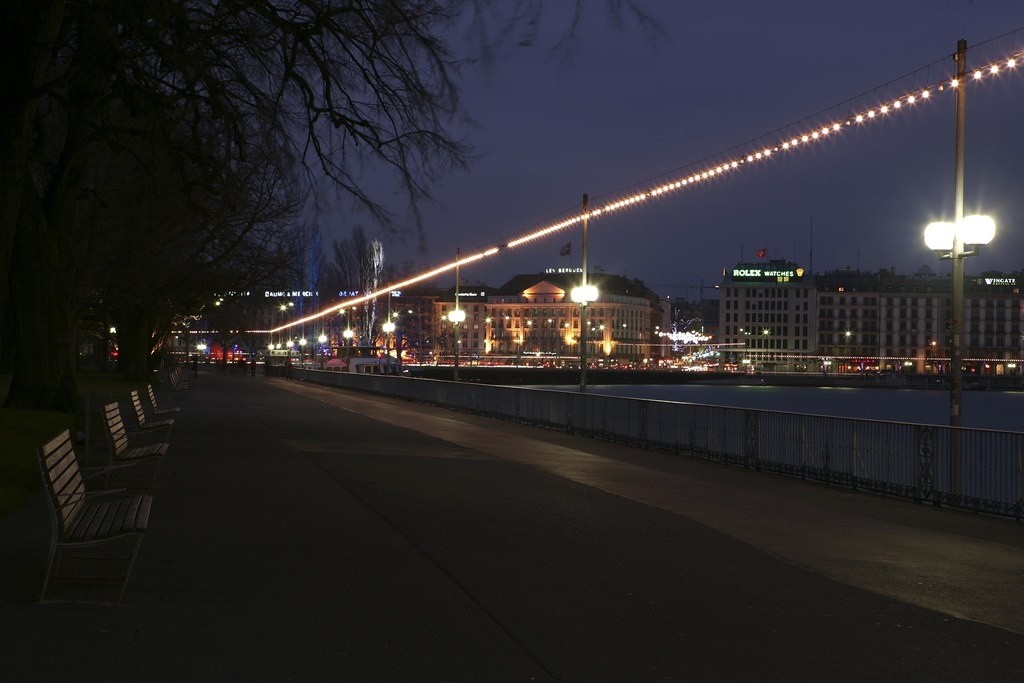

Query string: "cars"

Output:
[838,360,879,373]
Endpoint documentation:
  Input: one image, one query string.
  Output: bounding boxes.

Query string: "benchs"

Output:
[128,367,190,449]
[34,428,153,607]
[99,399,170,491]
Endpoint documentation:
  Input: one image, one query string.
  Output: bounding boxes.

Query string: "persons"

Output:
[192,354,199,378]
[287,363,293,380]
[250,358,255,377]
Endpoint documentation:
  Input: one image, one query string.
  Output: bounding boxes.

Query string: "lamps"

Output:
[923,214,997,260]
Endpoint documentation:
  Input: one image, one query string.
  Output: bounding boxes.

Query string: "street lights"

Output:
[446,308,467,381]
[298,336,308,363]
[382,321,396,355]
[343,328,356,372]
[919,210,1002,509]
[567,281,601,435]
[317,331,327,370]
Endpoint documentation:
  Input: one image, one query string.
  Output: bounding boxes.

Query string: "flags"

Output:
[757,249,765,258]
[560,244,571,256]
